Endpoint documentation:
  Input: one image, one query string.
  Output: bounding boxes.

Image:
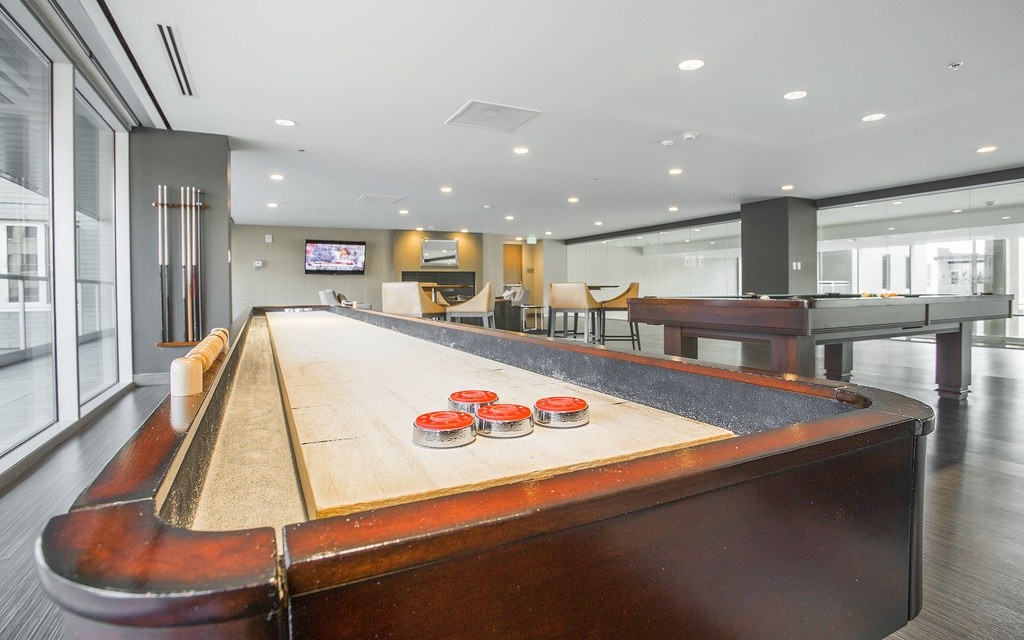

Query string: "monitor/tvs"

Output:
[303,239,367,276]
[421,238,460,268]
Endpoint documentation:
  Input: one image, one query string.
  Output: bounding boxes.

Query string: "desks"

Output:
[571,285,619,341]
[421,285,473,320]
[626,291,1014,400]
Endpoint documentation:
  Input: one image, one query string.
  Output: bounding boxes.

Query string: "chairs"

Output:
[319,290,372,311]
[382,282,642,351]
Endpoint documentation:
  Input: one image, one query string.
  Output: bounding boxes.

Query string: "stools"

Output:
[521,303,544,331]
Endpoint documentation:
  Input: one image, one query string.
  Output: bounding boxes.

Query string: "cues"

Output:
[157,182,205,342]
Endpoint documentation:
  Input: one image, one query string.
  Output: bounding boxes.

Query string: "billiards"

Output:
[760,295,770,299]
[862,289,896,297]
